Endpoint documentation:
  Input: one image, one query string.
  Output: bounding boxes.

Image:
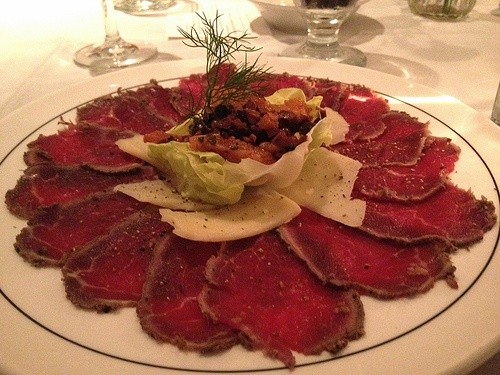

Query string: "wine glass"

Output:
[74,0,158,69]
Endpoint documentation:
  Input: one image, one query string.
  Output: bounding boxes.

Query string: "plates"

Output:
[1,58,500,374]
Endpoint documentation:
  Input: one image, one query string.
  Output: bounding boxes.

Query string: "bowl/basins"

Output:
[250,0,372,35]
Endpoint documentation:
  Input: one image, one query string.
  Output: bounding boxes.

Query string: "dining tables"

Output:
[0,0,499,375]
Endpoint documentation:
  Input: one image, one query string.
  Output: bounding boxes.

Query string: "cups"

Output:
[278,0,370,65]
[408,0,479,21]
